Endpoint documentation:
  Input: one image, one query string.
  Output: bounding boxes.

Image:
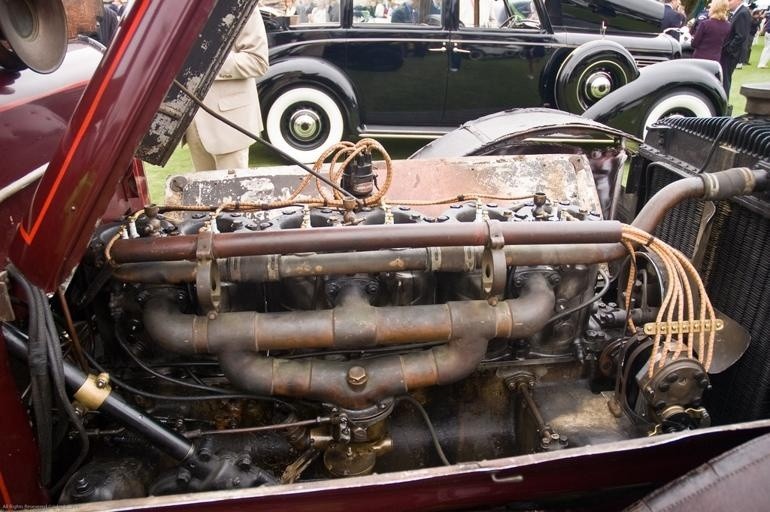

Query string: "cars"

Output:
[245,1,731,166]
[0,0,770,509]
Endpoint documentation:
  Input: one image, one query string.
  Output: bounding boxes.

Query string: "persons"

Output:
[181,4,269,172]
[660,0,770,103]
[92,9,118,47]
[114,0,125,16]
[296,1,535,27]
